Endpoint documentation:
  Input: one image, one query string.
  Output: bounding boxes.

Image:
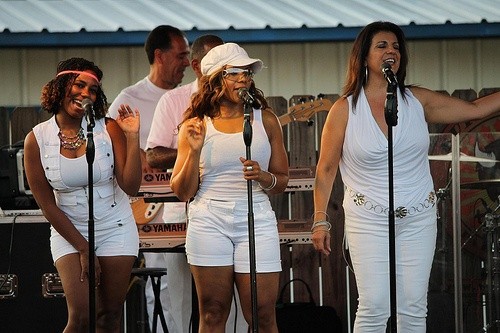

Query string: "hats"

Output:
[200,43,264,76]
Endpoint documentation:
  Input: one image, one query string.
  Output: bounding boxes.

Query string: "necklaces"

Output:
[58,128,86,149]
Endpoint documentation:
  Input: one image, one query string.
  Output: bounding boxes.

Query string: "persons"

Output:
[98,25,191,333]
[24,57,142,333]
[311,21,500,333]
[146,35,250,333]
[170,43,288,333]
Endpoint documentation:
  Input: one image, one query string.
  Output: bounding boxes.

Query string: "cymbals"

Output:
[428,152,499,162]
[460,179,500,189]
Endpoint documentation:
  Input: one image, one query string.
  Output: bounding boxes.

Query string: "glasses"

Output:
[222,68,253,77]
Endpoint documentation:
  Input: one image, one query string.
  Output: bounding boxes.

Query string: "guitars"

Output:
[130,94,333,224]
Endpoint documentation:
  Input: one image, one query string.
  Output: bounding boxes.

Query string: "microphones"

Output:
[380,62,397,87]
[238,87,261,110]
[82,98,95,127]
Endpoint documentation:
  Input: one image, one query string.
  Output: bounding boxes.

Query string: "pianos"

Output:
[136,218,314,252]
[129,166,316,198]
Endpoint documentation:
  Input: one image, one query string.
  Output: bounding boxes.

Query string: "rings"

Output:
[247,166,254,171]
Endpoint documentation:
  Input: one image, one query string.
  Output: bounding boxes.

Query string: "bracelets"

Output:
[311,211,332,233]
[258,172,277,191]
